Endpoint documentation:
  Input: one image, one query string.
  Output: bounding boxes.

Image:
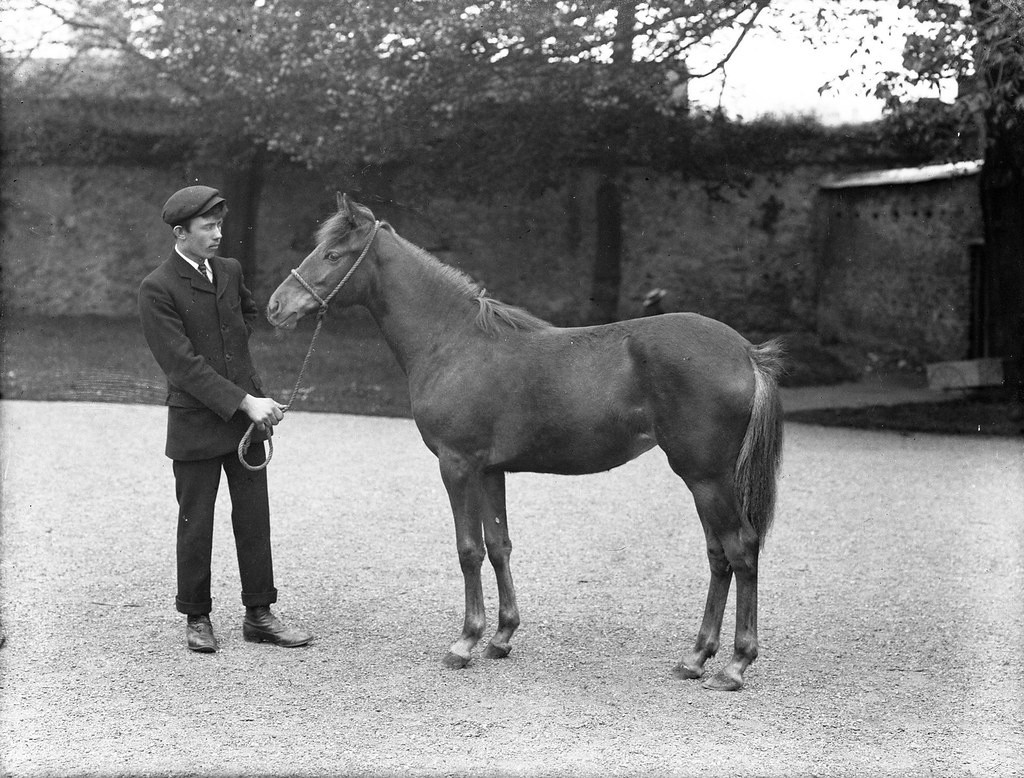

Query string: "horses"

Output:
[267,191,791,691]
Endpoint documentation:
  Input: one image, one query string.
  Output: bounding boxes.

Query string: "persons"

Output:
[643,289,668,317]
[138,185,313,652]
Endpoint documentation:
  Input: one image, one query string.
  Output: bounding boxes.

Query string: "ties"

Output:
[199,263,209,279]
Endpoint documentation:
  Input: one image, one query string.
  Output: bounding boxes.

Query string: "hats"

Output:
[162,185,225,225]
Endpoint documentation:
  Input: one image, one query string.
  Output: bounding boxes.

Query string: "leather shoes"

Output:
[187,613,218,652]
[243,608,313,647]
